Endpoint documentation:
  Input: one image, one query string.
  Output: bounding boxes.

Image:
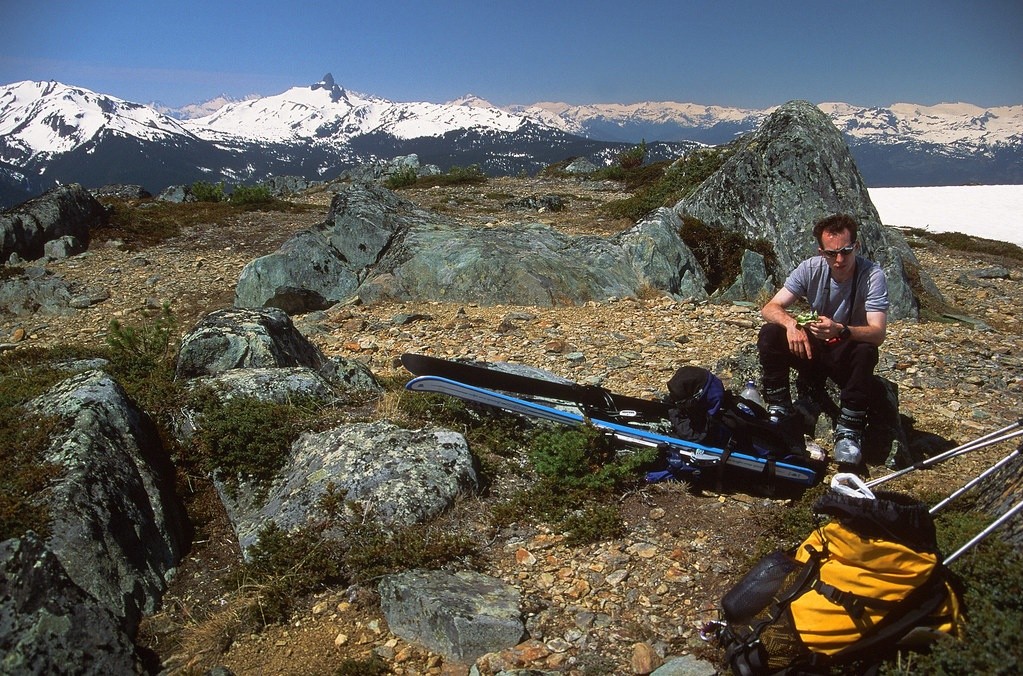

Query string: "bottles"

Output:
[738,381,760,416]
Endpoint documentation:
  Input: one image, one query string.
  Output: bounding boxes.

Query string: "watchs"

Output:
[836,322,851,339]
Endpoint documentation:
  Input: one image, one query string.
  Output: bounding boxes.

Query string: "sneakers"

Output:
[765,404,804,436]
[832,407,869,468]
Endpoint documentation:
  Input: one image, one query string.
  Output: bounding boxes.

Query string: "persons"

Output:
[756,213,890,467]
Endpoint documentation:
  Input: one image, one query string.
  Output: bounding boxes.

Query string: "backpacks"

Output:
[702,471,966,676]
[646,365,821,480]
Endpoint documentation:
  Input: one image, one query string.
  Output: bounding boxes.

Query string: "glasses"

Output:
[822,242,856,259]
